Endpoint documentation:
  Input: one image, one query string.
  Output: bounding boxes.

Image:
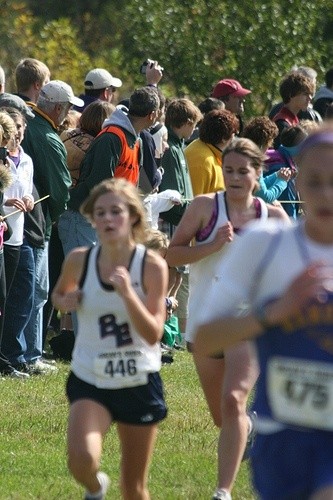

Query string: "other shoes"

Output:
[9,369,29,378]
[85,472,109,500]
[242,411,257,462]
[30,359,57,373]
[211,488,231,500]
[14,363,43,376]
[40,358,56,365]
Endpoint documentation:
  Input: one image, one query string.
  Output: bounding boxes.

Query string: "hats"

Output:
[39,80,85,107]
[0,92,36,119]
[212,79,252,99]
[84,68,122,89]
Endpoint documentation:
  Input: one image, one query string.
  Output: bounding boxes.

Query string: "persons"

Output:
[52,178,169,499]
[0,52,332,377]
[166,139,292,500]
[193,132,332,500]
